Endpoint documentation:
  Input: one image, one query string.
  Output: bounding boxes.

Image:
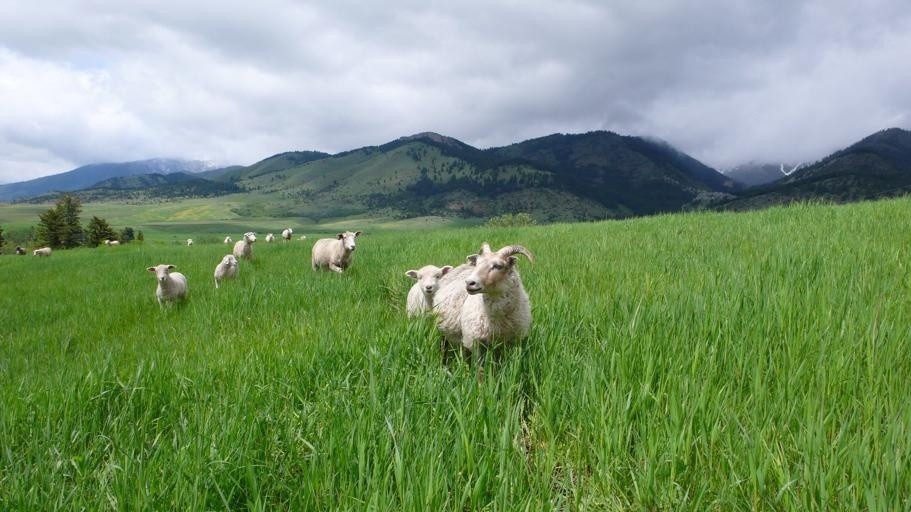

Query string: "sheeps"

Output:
[280,228,293,244]
[265,233,276,242]
[232,232,256,261]
[404,263,453,320]
[33,247,52,257]
[212,254,238,290]
[147,264,189,311]
[310,230,361,274]
[15,246,26,256]
[434,239,539,371]
